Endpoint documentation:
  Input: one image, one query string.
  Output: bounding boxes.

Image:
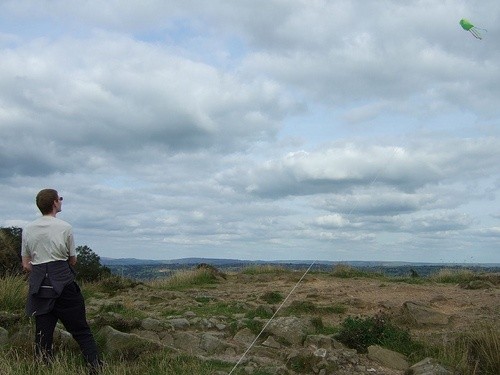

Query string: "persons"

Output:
[20,189,98,372]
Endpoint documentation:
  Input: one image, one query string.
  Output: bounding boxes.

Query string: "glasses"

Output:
[57,197,63,200]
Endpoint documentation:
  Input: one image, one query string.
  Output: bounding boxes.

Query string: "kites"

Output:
[457,16,490,42]
